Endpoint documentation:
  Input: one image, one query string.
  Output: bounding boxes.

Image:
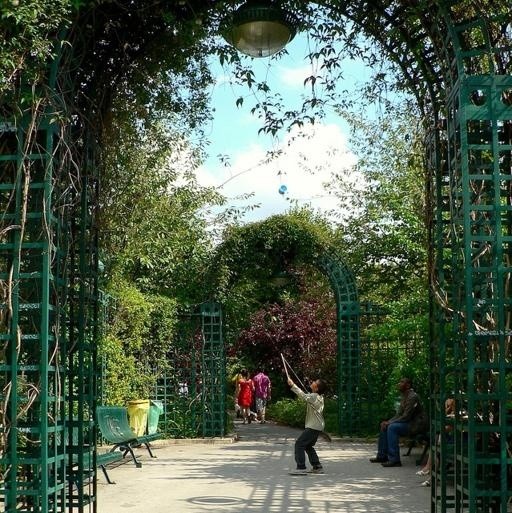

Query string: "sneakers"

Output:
[308,467,324,474]
[381,460,402,467]
[369,457,388,462]
[287,469,307,475]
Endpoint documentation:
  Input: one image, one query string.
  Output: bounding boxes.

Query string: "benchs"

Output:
[54,415,122,482]
[96,405,163,466]
[402,418,442,465]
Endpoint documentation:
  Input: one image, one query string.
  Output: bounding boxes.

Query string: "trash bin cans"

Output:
[127,400,164,438]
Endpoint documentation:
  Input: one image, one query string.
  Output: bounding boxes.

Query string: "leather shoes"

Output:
[415,469,430,477]
[421,480,430,487]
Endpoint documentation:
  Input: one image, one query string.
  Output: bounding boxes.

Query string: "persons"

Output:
[252,369,271,424]
[236,369,256,424]
[416,399,460,486]
[196,374,207,399]
[229,368,244,418]
[179,379,188,397]
[287,377,327,475]
[370,378,426,468]
